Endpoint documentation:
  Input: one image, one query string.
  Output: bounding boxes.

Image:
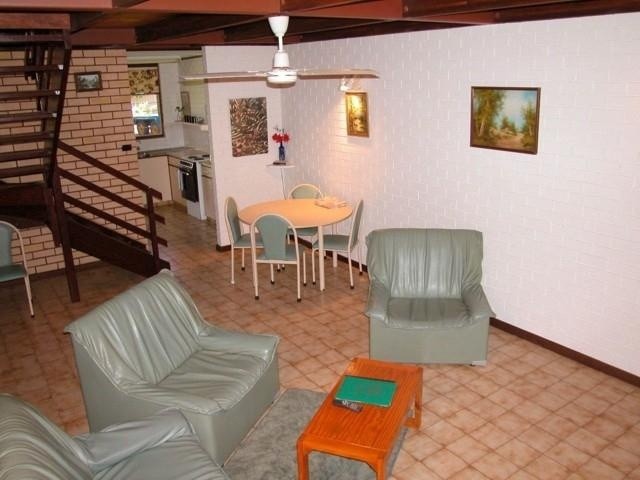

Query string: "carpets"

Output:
[223,387,413,479]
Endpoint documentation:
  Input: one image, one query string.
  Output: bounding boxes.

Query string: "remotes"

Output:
[332,399,362,413]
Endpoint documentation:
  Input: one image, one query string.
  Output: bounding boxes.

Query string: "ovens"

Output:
[179,160,198,203]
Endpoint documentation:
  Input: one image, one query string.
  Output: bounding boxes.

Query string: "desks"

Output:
[237,198,354,291]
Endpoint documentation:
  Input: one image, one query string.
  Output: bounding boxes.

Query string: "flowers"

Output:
[271,127,290,144]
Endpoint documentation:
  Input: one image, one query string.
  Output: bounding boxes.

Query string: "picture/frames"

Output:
[74,71,102,92]
[469,86,541,154]
[346,92,369,137]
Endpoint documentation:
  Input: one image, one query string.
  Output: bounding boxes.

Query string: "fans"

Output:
[177,15,380,87]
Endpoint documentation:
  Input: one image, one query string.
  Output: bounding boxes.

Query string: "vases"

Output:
[279,144,285,161]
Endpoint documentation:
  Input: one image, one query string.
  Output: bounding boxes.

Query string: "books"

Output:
[334,375,398,408]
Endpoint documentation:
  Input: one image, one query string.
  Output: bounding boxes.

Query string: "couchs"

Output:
[1,393,235,480]
[364,227,496,369]
[61,272,280,466]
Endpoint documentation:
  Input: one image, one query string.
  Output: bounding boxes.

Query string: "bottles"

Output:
[147,123,152,134]
[184,115,201,123]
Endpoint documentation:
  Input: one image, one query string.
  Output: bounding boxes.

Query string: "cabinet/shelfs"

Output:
[201,162,216,225]
[168,154,186,207]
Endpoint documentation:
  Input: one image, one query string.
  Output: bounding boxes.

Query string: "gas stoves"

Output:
[183,152,210,162]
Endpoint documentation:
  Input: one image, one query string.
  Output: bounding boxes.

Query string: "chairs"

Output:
[311,199,364,289]
[283,183,324,264]
[250,213,307,302]
[225,196,280,284]
[0,221,35,317]
